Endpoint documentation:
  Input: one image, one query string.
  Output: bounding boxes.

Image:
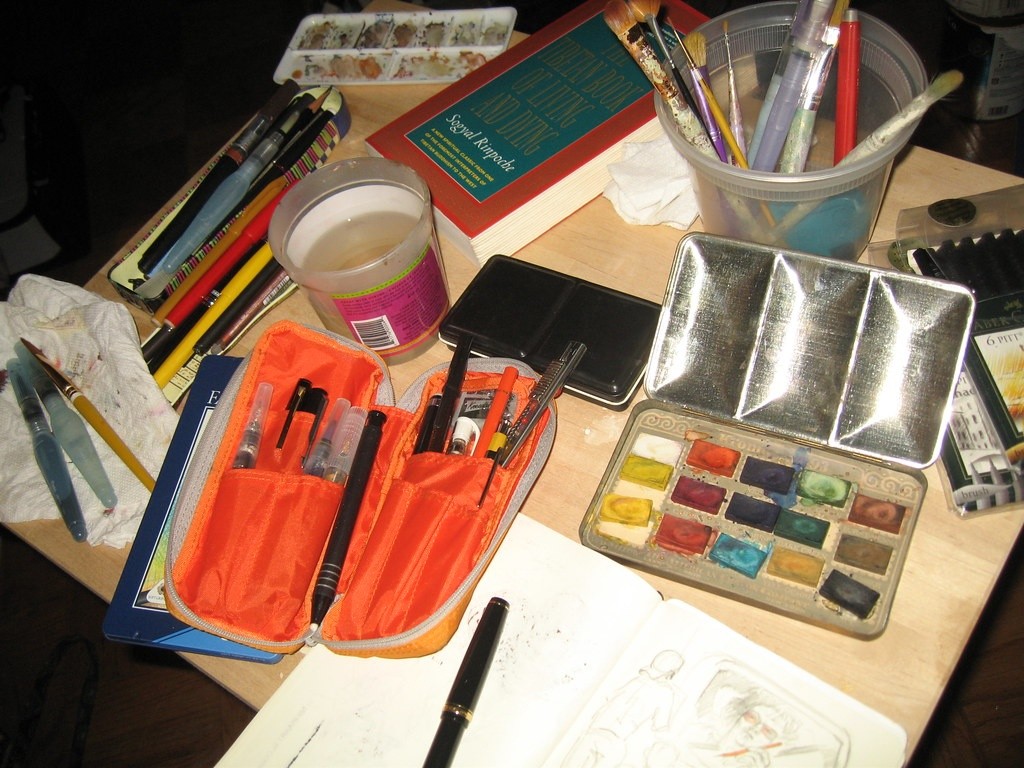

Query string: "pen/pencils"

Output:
[309,409,387,631]
[232,382,274,468]
[445,416,481,457]
[296,388,329,469]
[276,378,313,449]
[746,0,860,222]
[475,413,514,509]
[136,79,337,391]
[412,393,442,454]
[302,397,352,478]
[323,405,368,485]
[427,334,472,452]
[471,365,519,458]
[421,597,509,768]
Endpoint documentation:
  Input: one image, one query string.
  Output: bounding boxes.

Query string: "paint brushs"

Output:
[767,71,964,246]
[20,339,155,493]
[604,0,787,250]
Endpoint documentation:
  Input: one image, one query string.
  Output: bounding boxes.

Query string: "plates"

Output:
[274,6,518,86]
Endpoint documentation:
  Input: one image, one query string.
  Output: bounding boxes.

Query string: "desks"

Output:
[0,0,1024,768]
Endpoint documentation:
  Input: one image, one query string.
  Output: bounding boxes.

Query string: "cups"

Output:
[654,0,929,262]
[267,156,452,366]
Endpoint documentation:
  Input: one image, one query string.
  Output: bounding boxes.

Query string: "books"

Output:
[366,0,758,267]
